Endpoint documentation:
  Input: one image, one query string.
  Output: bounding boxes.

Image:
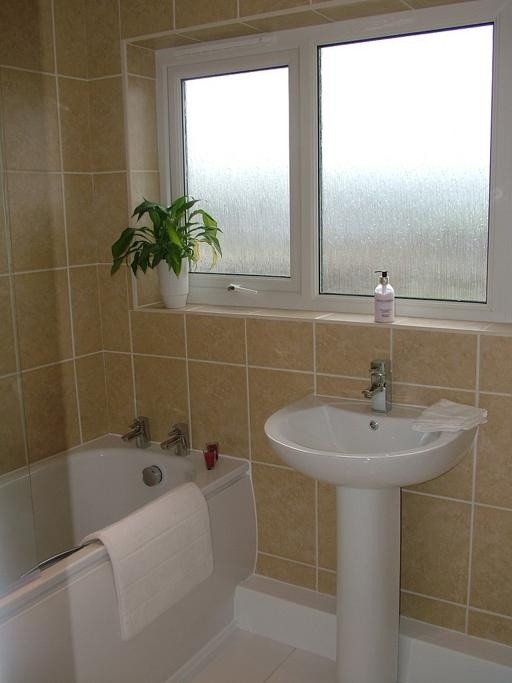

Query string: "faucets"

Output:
[121,415,150,450]
[160,423,190,458]
[363,359,392,412]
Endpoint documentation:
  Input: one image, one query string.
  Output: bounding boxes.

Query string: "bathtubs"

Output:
[0,434,256,682]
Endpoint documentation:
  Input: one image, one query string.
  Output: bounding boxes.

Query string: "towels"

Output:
[410,398,488,433]
[79,481,214,640]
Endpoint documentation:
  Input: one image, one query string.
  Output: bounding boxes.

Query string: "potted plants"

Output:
[111,195,224,309]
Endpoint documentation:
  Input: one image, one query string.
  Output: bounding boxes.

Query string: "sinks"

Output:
[265,393,477,487]
